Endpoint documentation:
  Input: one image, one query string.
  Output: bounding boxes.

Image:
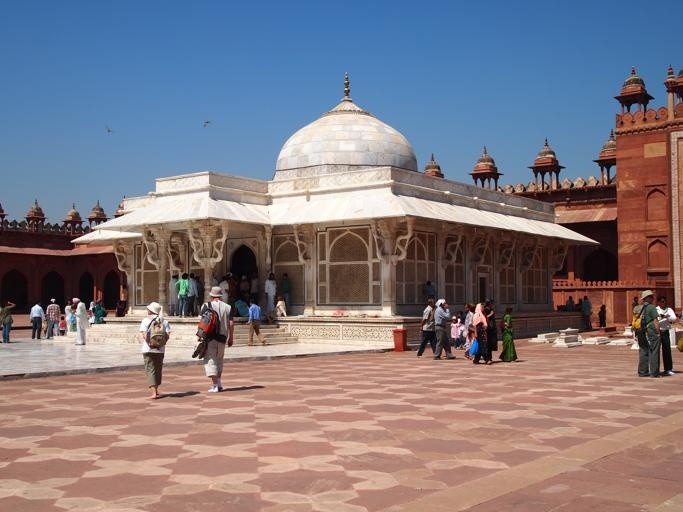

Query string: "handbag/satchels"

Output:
[469,339,478,355]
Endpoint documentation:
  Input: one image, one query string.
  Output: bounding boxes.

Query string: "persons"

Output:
[566,293,606,331]
[195,287,234,393]
[630,289,663,378]
[166,269,291,325]
[632,297,638,309]
[416,280,518,365]
[0,301,15,342]
[654,294,677,376]
[29,298,107,345]
[245,299,267,346]
[115,284,128,317]
[139,302,171,398]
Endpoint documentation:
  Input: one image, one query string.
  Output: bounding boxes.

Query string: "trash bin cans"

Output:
[393,329,408,352]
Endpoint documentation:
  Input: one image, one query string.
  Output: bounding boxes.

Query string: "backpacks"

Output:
[147,317,168,350]
[195,302,219,340]
[632,303,651,333]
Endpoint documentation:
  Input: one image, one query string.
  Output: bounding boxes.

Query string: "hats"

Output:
[639,290,656,300]
[146,302,161,315]
[209,286,223,298]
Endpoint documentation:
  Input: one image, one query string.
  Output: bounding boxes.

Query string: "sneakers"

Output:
[650,369,675,378]
[192,341,208,359]
